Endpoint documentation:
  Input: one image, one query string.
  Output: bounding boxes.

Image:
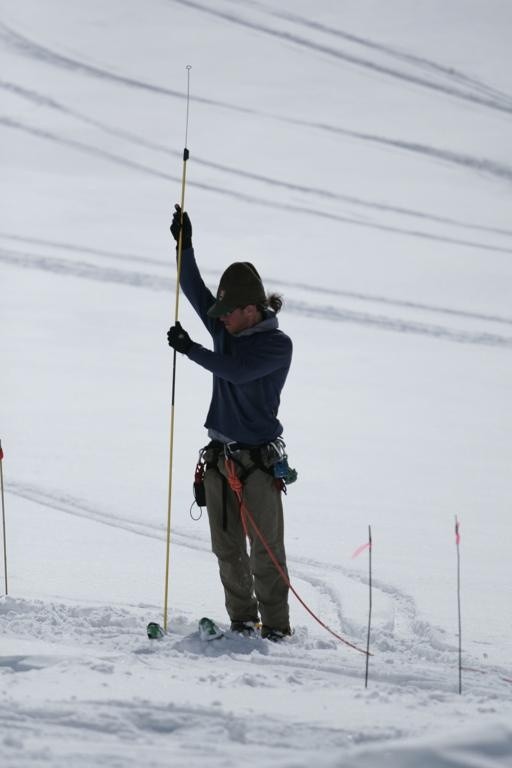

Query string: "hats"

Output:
[205,262,265,320]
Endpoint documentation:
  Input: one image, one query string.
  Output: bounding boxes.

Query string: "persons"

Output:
[165,200,297,644]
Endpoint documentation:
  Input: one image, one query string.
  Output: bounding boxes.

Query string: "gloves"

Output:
[170,203,193,248]
[167,320,194,355]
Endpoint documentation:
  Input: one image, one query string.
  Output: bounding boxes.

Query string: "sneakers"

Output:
[229,618,259,636]
